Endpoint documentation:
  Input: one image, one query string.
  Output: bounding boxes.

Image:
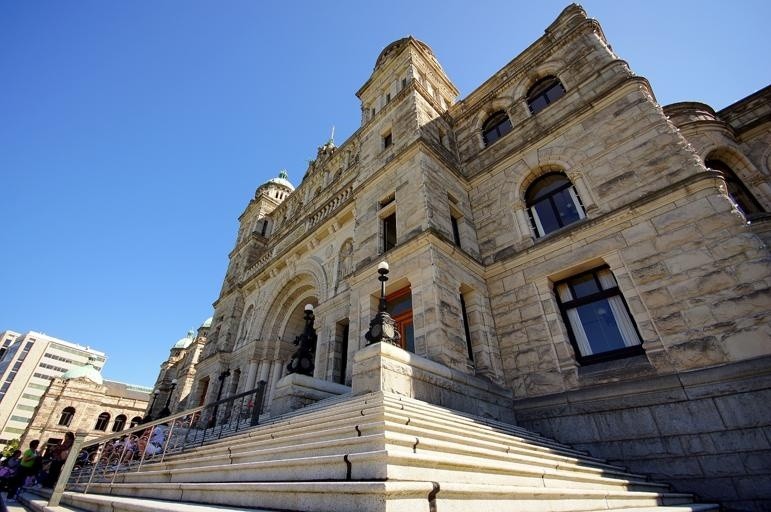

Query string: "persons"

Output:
[0,418,164,503]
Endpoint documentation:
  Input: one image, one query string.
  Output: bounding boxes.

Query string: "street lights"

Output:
[142,377,176,422]
[363,261,402,348]
[283,303,317,375]
